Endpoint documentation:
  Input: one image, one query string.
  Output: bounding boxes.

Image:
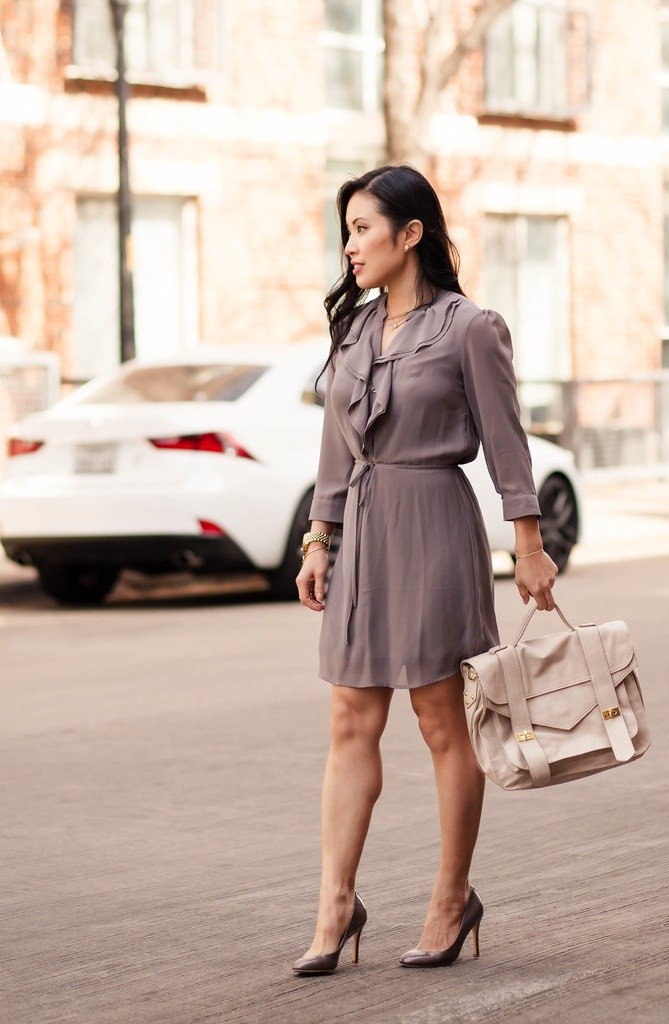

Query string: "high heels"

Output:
[293,893,367,974]
[398,887,485,967]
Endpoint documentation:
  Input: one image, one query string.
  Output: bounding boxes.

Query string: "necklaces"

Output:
[387,294,431,328]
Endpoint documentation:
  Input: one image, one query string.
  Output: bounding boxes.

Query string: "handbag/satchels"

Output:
[461,598,651,791]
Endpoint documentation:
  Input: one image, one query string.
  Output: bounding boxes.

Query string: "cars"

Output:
[0,342,587,607]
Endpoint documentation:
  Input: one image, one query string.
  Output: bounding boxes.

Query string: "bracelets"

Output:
[304,547,329,559]
[303,532,330,556]
[515,548,543,559]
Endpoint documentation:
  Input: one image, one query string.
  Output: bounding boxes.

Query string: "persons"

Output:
[292,162,558,972]
